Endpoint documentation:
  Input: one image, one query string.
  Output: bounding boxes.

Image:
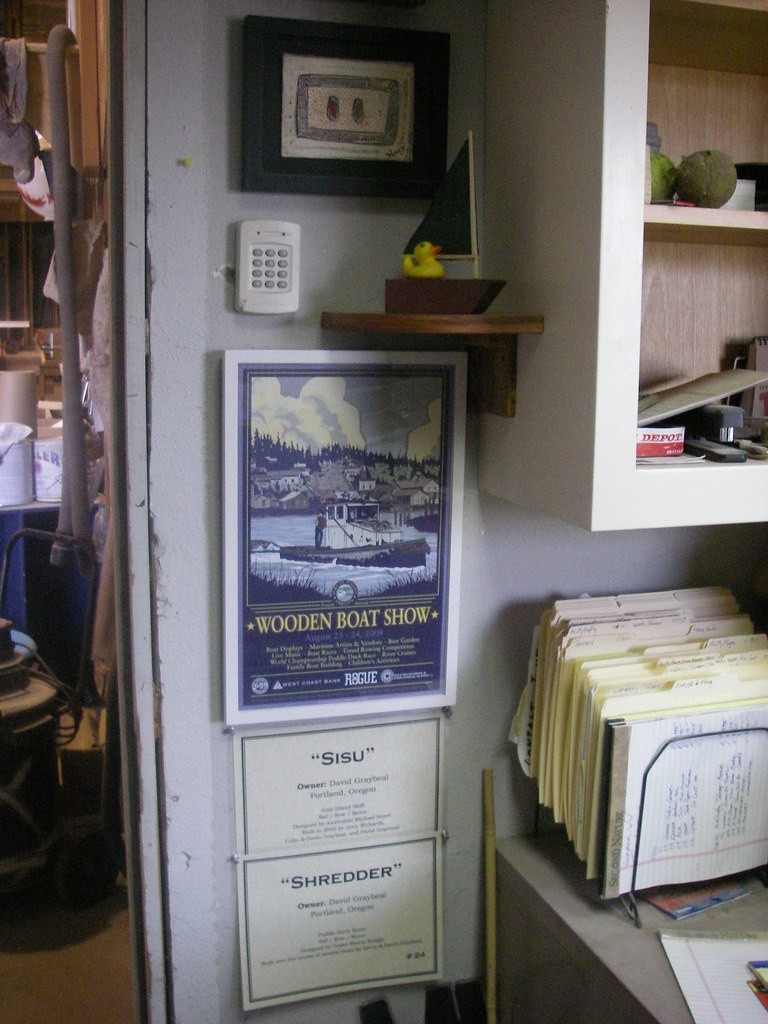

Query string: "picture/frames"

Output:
[221,348,468,725]
[240,15,452,199]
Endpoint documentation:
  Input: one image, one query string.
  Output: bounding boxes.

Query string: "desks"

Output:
[0,501,99,705]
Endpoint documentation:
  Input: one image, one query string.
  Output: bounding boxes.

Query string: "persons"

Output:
[314,512,328,548]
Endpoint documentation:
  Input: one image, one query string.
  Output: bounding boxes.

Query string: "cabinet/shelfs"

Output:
[476,0,768,532]
[478,829,768,1024]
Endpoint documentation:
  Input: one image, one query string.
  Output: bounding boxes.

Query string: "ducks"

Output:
[402,241,446,279]
